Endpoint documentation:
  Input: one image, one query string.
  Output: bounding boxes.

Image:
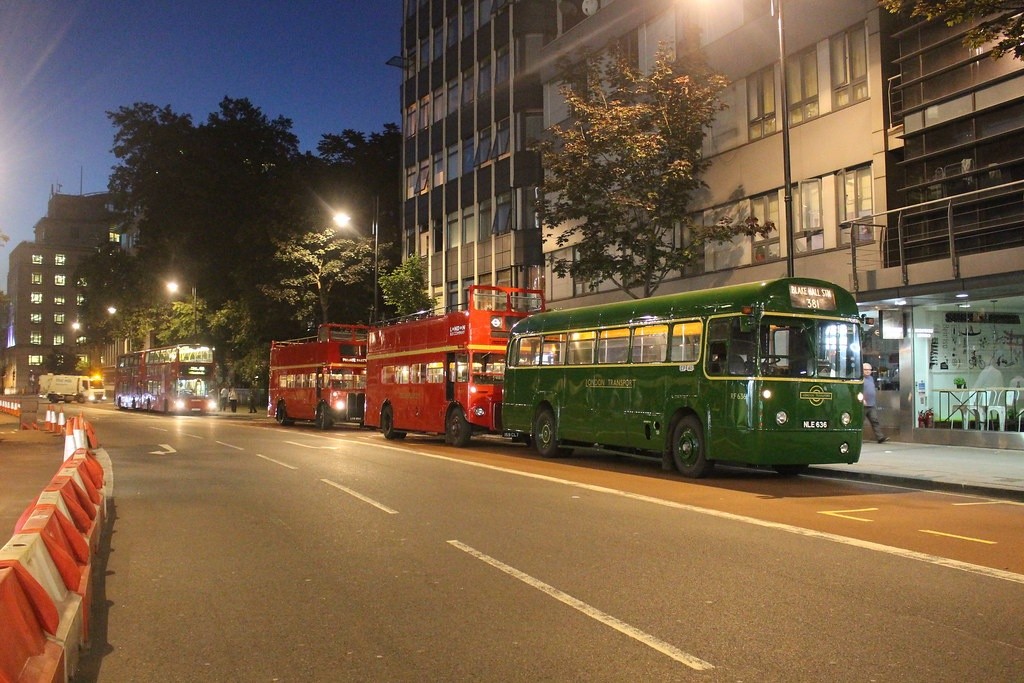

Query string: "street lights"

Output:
[167,281,197,344]
[333,196,378,324]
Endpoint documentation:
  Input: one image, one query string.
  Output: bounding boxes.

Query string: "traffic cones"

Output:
[0,397,21,417]
[77,410,89,450]
[48,405,58,433]
[63,420,78,463]
[73,417,84,451]
[53,407,66,436]
[41,403,51,431]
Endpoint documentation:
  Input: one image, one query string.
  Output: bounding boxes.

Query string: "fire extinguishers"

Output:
[925,407,934,428]
[918,410,925,428]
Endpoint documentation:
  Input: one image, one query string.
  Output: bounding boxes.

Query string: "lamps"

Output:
[991,300,997,334]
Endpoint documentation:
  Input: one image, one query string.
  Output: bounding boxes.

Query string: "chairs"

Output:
[950,388,1019,430]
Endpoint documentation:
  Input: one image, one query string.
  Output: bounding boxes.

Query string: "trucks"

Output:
[39,373,106,404]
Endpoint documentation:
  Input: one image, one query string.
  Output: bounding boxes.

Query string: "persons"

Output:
[863,363,890,443]
[710,349,721,372]
[327,375,364,388]
[248,389,257,413]
[177,381,202,397]
[218,385,229,412]
[148,352,169,362]
[390,374,443,383]
[461,368,494,383]
[228,387,238,413]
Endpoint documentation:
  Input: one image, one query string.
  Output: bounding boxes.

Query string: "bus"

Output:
[501,278,860,480]
[363,285,546,448]
[267,325,376,431]
[113,344,220,417]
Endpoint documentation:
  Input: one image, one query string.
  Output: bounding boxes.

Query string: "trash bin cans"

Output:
[19,400,38,426]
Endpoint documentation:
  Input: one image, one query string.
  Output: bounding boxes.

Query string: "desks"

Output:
[929,387,1024,432]
